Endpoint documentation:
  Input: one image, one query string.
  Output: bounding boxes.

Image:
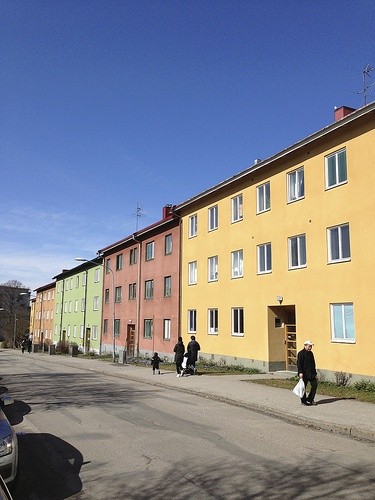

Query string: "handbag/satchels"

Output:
[292,376,305,398]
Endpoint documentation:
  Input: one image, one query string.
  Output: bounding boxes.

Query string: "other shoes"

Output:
[307,398,317,405]
[194,369,197,375]
[181,371,184,376]
[301,401,309,406]
[177,375,180,377]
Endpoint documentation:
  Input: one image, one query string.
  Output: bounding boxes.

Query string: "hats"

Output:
[304,341,314,346]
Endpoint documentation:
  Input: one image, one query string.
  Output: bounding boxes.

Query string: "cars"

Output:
[0,382,19,485]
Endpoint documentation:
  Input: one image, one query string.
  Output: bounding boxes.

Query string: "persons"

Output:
[151,352,163,375]
[297,341,317,406]
[186,336,200,375]
[21,339,31,354]
[173,337,185,377]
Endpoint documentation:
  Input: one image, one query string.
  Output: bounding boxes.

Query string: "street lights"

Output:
[19,291,46,355]
[0,307,16,347]
[74,256,116,362]
